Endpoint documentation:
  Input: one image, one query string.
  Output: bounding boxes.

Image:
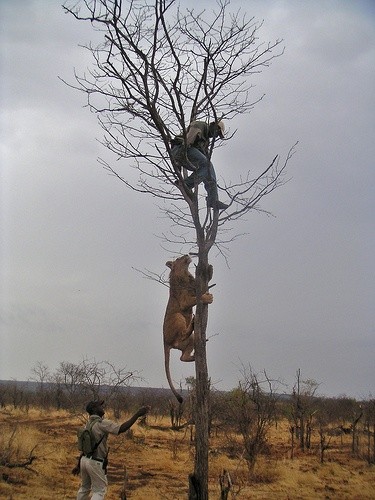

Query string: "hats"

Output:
[213,121,225,140]
[87,400,105,413]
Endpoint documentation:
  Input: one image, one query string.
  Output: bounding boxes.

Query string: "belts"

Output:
[82,454,103,463]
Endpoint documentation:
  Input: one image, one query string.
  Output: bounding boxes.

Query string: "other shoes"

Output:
[208,201,229,209]
[176,178,195,202]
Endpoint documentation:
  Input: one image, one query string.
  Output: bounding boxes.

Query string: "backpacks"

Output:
[77,418,107,456]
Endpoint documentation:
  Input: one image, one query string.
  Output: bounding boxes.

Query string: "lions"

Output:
[162,255,214,405]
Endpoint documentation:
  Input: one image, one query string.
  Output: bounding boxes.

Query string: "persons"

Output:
[70,397,152,500]
[169,119,230,210]
